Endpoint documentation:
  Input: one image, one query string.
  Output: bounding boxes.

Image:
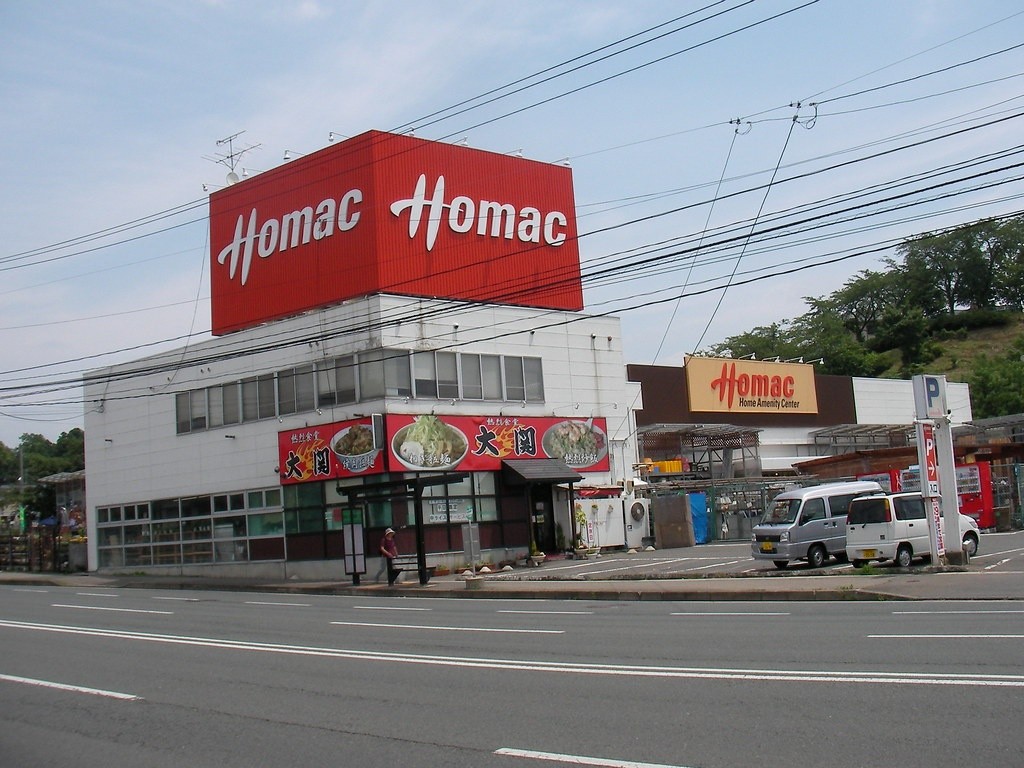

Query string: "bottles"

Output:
[901,470,921,481]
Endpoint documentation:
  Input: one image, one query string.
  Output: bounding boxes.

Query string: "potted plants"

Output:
[530,551,546,563]
[574,543,601,555]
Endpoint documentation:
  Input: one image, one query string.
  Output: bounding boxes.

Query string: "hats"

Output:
[385,528,394,536]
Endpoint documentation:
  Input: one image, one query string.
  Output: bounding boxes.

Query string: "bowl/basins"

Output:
[391,421,469,471]
[330,423,381,473]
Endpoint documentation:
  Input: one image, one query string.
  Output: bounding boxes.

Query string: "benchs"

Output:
[391,556,436,571]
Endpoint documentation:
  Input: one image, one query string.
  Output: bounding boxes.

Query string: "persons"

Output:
[380,527,403,587]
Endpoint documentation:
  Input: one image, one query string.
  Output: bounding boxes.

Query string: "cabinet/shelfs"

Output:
[124,530,213,566]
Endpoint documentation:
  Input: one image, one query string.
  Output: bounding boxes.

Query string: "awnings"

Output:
[502,458,583,483]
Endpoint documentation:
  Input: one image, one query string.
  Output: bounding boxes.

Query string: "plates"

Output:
[541,420,608,468]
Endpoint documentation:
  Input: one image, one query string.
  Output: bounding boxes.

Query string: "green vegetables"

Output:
[405,416,465,457]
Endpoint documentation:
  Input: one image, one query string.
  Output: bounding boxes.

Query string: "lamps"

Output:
[277,396,619,426]
[737,353,824,364]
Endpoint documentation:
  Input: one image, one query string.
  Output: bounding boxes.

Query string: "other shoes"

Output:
[395,581,403,584]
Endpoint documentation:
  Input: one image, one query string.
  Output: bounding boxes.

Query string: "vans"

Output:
[845,490,981,570]
[749,479,888,569]
